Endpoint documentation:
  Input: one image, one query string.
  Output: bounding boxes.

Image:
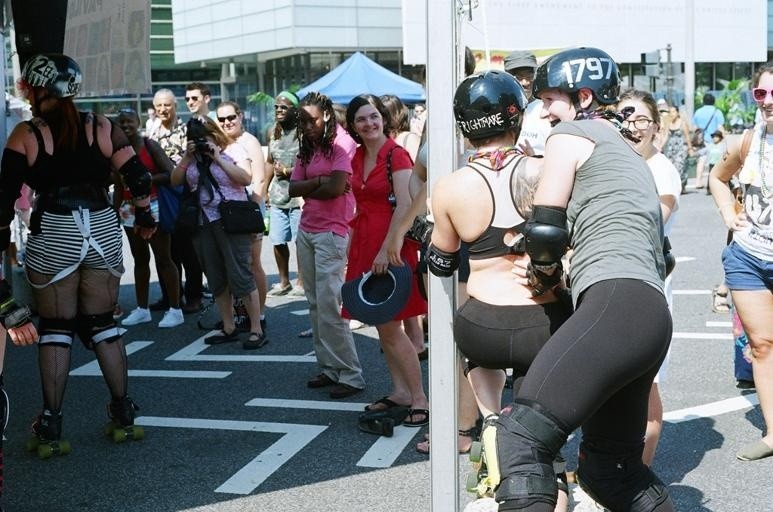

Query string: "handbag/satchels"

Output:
[692,128,706,147]
[217,197,266,235]
[158,187,199,234]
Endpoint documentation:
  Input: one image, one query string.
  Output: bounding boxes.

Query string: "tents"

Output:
[295,53,425,113]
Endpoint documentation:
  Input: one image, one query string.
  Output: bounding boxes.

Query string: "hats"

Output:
[711,130,723,138]
[504,51,539,73]
[342,260,412,326]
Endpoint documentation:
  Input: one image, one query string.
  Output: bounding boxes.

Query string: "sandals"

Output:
[712,284,732,315]
[416,430,476,455]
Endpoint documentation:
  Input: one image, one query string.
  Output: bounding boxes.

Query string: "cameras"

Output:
[193,135,210,156]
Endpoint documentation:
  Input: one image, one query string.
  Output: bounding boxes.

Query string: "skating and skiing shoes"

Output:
[466,437,493,499]
[26,409,73,460]
[104,395,146,444]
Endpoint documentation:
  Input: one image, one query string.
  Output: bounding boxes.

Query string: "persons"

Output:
[2,53,146,460]
[658,59,773,462]
[396,47,683,512]
[113,84,267,349]
[264,92,429,427]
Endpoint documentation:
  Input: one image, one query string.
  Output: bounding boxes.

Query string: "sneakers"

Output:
[329,381,360,398]
[243,331,268,349]
[235,315,267,330]
[158,306,185,328]
[121,307,152,327]
[736,440,770,462]
[307,374,333,388]
[204,327,239,345]
[271,283,292,296]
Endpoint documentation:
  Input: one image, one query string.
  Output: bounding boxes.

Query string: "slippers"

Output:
[402,406,429,428]
[365,395,411,413]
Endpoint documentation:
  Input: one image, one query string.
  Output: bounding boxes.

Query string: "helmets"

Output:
[453,69,528,139]
[21,54,83,99]
[533,46,624,104]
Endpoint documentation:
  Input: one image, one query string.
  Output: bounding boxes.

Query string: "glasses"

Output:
[751,87,772,103]
[218,114,237,123]
[622,118,655,131]
[118,108,132,114]
[274,105,289,110]
[185,96,199,102]
